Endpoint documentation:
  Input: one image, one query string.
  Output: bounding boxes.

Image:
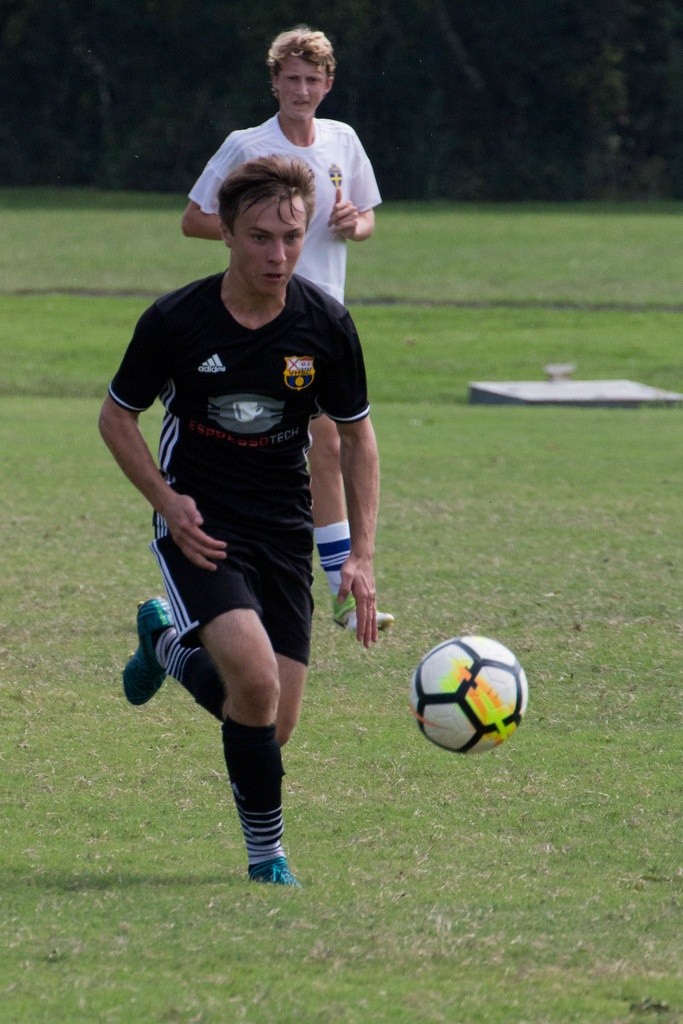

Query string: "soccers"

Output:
[410,636,529,754]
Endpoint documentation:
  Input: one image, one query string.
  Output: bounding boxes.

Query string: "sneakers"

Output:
[331,604,395,631]
[248,857,301,890]
[122,596,175,705]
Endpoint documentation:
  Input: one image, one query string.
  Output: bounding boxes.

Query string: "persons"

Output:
[180,23,396,629]
[99,155,381,889]
